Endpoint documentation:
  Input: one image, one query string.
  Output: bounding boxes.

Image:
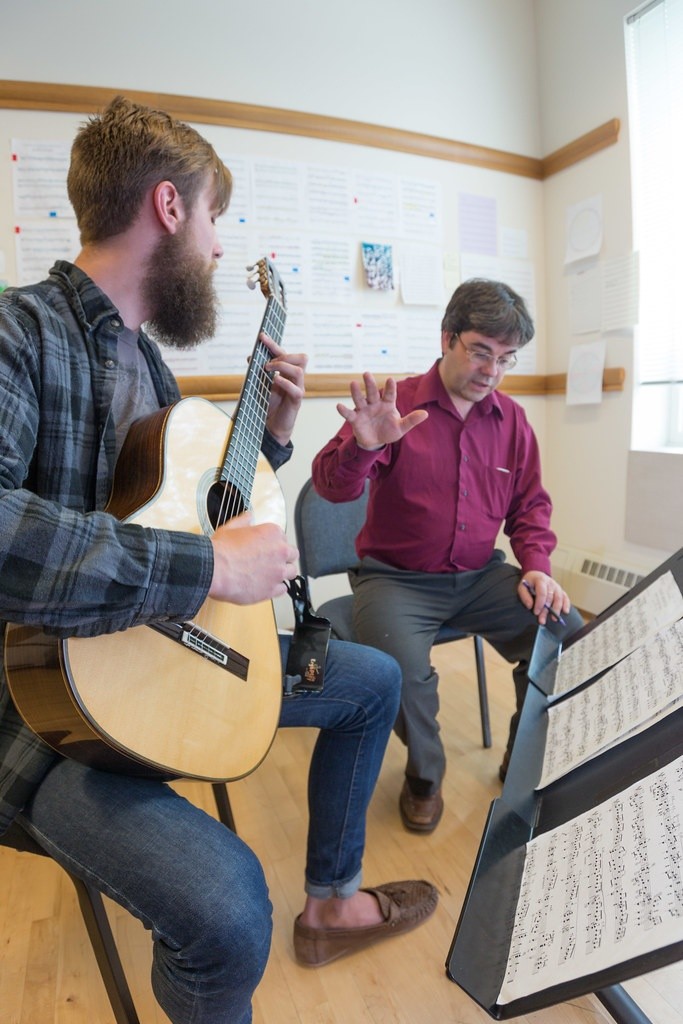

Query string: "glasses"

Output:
[453,331,518,370]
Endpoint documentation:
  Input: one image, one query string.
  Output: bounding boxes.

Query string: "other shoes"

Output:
[294,879,437,966]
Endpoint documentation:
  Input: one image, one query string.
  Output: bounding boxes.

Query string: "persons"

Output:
[2,94,439,1024]
[314,279,586,833]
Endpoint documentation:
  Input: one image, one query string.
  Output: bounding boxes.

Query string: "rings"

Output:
[548,591,553,594]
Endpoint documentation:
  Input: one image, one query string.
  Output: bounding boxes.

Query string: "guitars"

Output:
[3,259,291,785]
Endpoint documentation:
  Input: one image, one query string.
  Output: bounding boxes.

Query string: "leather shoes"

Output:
[498,753,512,780]
[398,775,446,830]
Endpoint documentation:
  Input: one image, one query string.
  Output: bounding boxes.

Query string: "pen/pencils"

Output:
[521,579,566,627]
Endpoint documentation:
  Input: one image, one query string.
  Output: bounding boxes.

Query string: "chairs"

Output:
[0,775,240,1024]
[295,472,494,749]
